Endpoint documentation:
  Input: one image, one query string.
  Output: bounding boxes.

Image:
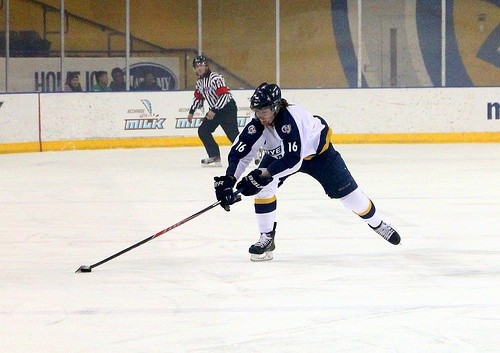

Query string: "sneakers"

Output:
[200,156,222,167]
[249,222,277,261]
[367,220,402,246]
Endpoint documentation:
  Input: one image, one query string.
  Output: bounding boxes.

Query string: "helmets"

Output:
[250,82,281,113]
[192,56,208,66]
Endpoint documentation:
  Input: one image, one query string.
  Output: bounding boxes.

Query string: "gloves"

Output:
[214,176,242,212]
[236,169,273,196]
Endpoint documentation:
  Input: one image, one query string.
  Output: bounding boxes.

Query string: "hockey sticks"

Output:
[75,189,243,273]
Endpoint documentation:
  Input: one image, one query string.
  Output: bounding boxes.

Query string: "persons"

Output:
[188,56,240,167]
[213,81,400,262]
[63,68,163,92]
